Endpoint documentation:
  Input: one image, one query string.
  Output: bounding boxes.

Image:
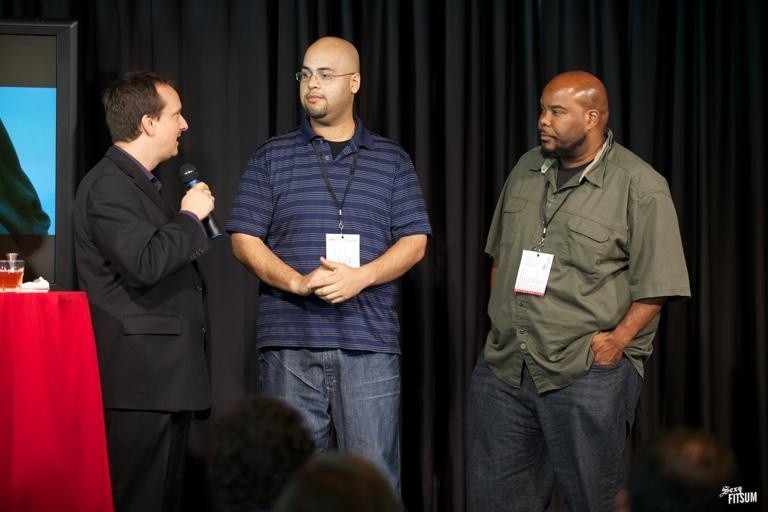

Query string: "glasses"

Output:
[294,69,357,82]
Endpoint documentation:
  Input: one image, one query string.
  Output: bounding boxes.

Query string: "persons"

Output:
[270,447,404,512]
[223,34,435,512]
[0,112,52,280]
[608,421,740,512]
[70,72,222,511]
[455,69,694,509]
[201,393,318,511]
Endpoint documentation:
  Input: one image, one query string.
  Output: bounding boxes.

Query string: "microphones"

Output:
[180,165,223,240]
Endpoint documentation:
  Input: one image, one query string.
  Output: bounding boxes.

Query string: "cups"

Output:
[0,260,25,294]
